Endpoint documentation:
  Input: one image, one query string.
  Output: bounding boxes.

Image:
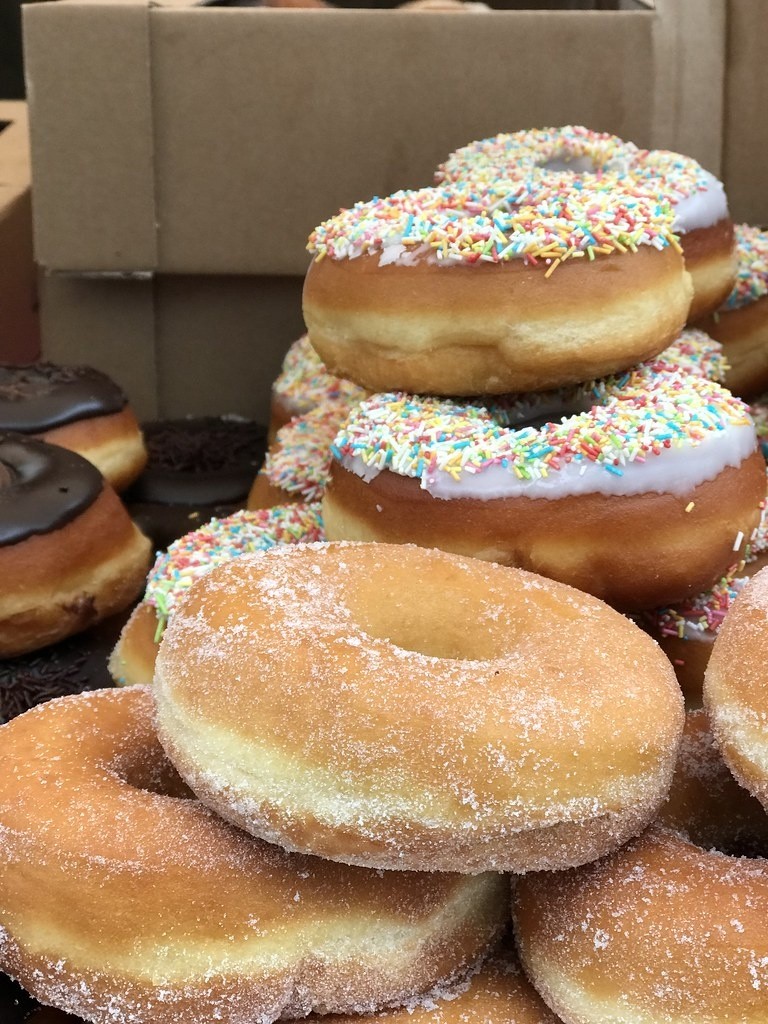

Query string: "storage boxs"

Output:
[22,0,661,426]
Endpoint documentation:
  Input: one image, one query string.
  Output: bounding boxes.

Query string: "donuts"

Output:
[0,124,768,1024]
